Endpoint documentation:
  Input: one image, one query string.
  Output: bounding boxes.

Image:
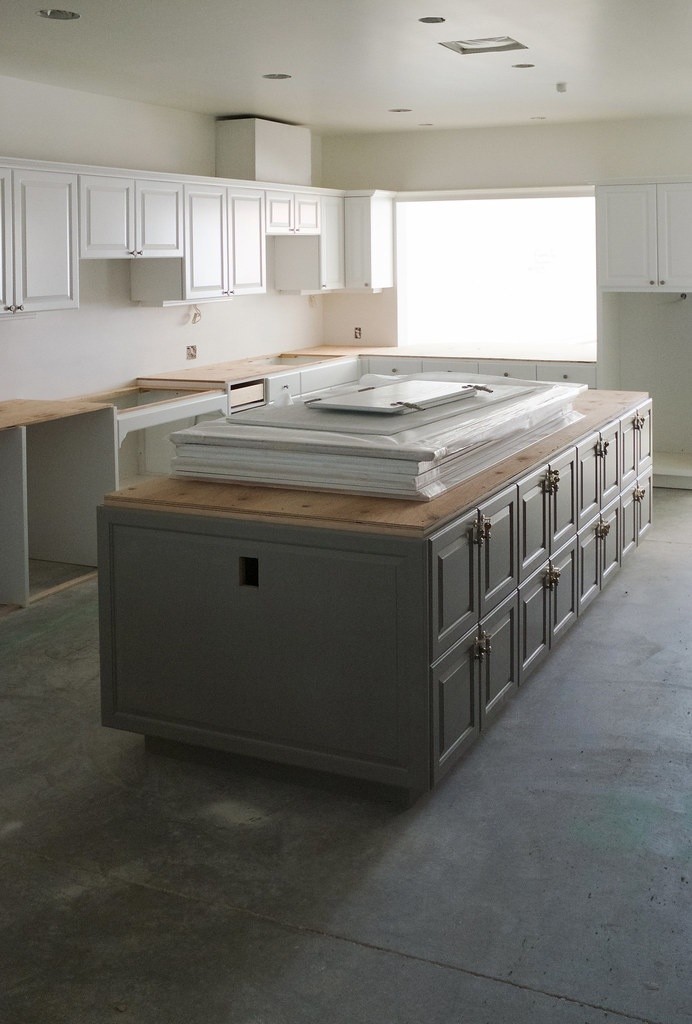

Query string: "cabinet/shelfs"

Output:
[137,357,598,478]
[584,174,692,293]
[94,372,655,813]
[0,155,397,315]
